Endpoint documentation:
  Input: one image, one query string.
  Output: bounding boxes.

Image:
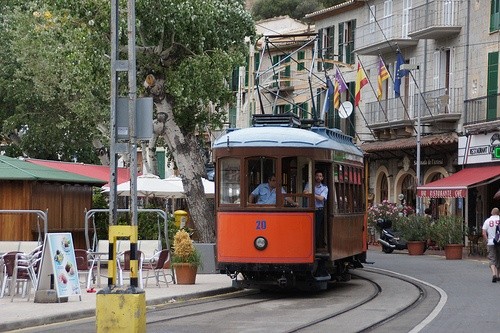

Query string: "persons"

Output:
[303,171,328,249]
[249,174,298,205]
[481,207,500,283]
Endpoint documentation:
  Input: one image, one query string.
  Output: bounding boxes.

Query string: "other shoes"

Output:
[496,277,500,280]
[492,275,496,282]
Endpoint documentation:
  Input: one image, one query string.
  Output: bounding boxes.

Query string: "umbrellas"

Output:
[104,173,237,210]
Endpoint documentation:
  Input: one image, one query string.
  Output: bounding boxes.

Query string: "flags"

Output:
[323,77,334,113]
[378,59,390,102]
[394,52,410,98]
[334,69,348,111]
[355,63,368,107]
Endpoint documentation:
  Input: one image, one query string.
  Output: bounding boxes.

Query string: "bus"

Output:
[210,112,370,297]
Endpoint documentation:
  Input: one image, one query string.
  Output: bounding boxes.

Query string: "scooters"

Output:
[376,218,407,253]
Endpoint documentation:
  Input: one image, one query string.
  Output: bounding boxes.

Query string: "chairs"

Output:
[74,248,176,292]
[0,244,43,303]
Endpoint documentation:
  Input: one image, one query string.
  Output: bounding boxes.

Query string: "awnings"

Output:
[416,166,500,246]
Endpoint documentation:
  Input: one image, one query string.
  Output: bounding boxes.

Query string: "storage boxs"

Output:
[377,218,393,229]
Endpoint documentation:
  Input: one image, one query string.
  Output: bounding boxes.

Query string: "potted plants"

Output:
[396,213,468,260]
[170,229,201,285]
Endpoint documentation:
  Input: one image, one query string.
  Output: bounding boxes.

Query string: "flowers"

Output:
[366,201,413,234]
